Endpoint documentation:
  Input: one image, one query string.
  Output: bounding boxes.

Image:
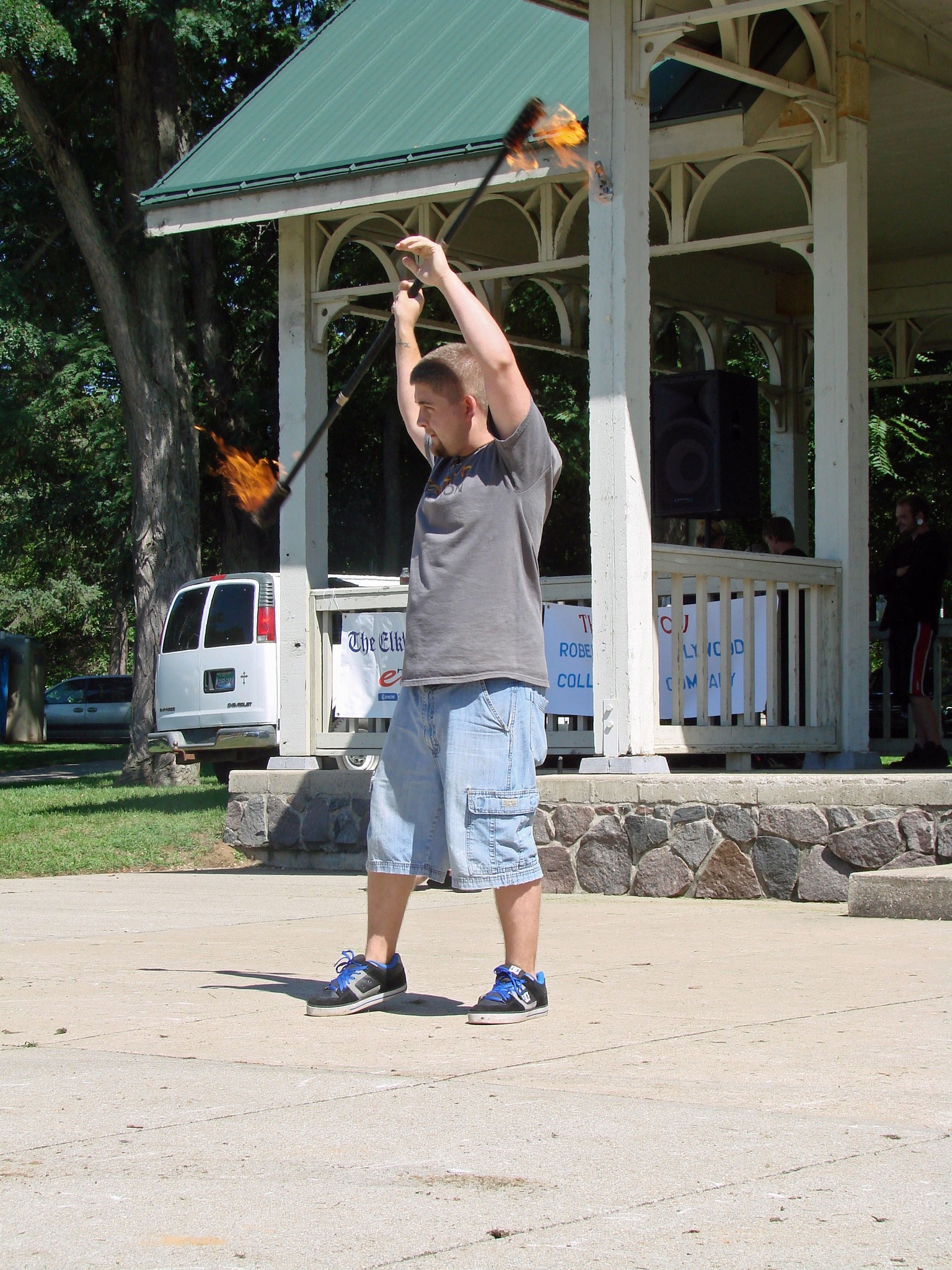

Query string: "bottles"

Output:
[400,567,409,585]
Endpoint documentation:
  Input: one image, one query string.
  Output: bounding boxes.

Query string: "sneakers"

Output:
[467,964,548,1024]
[306,950,407,1016]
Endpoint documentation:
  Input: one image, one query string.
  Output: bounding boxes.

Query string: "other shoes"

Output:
[890,744,950,769]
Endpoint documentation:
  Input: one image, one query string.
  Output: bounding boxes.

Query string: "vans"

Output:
[145,572,413,784]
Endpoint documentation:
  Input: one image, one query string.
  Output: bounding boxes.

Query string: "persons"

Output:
[745,517,809,555]
[696,520,723,548]
[306,234,560,1026]
[874,495,950,769]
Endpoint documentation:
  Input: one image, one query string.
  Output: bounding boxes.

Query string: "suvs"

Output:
[43,674,136,742]
